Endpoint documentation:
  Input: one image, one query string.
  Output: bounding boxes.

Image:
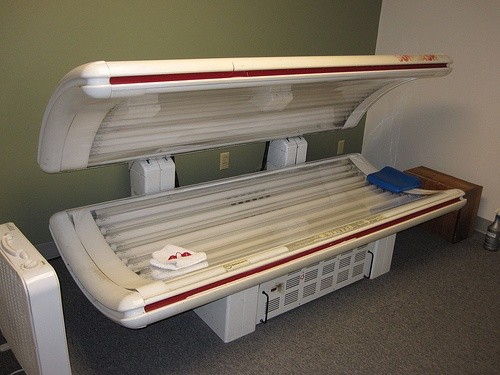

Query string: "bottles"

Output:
[483,209,500,252]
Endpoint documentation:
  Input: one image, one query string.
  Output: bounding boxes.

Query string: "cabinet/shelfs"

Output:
[403,165,484,246]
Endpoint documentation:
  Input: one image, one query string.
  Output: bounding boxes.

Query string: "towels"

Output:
[148,243,207,271]
[148,261,209,280]
